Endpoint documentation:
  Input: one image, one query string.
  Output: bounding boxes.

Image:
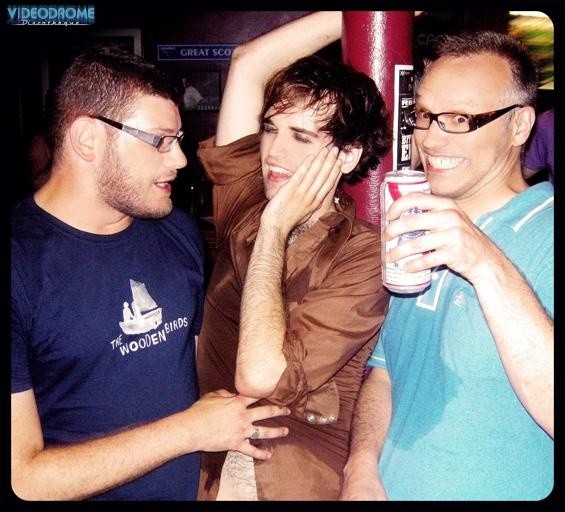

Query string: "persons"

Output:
[521,108,555,186]
[193,11,425,501]
[338,30,555,501]
[11,46,293,501]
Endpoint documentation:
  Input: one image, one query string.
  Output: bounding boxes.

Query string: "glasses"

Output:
[403,103,523,133]
[92,113,186,152]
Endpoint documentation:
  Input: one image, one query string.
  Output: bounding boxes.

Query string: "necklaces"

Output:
[287,220,313,243]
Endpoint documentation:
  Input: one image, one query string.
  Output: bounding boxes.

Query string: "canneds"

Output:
[380,170,431,294]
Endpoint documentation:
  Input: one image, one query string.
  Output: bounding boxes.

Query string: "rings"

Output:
[251,426,260,440]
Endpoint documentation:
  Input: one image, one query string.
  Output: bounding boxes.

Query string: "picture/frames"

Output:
[41,27,144,120]
[168,69,222,113]
